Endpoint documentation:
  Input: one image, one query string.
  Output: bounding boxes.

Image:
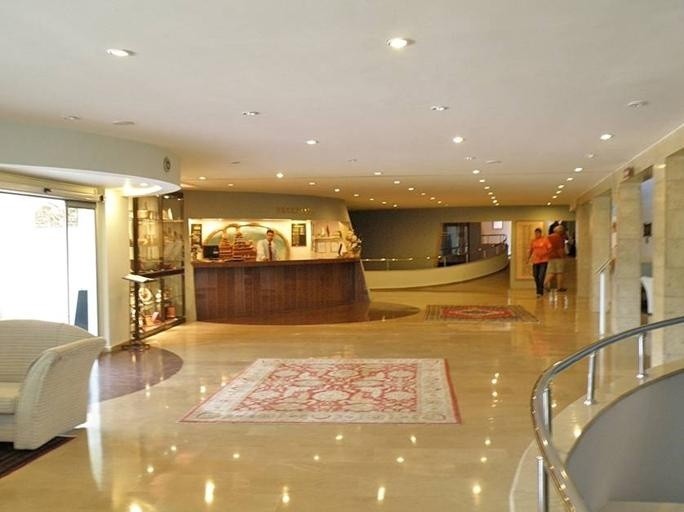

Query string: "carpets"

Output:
[0,436,76,479]
[423,304,539,324]
[174,357,463,425]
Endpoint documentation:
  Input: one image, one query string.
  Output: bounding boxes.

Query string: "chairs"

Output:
[0,319,107,451]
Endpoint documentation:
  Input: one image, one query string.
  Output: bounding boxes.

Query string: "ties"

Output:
[269,240,272,260]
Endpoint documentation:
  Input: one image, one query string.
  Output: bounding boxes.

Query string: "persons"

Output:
[256,230,279,262]
[525,226,568,298]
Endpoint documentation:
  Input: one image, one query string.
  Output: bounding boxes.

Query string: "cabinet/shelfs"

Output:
[128,191,185,339]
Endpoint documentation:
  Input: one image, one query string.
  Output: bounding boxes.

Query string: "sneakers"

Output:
[536,287,568,298]
